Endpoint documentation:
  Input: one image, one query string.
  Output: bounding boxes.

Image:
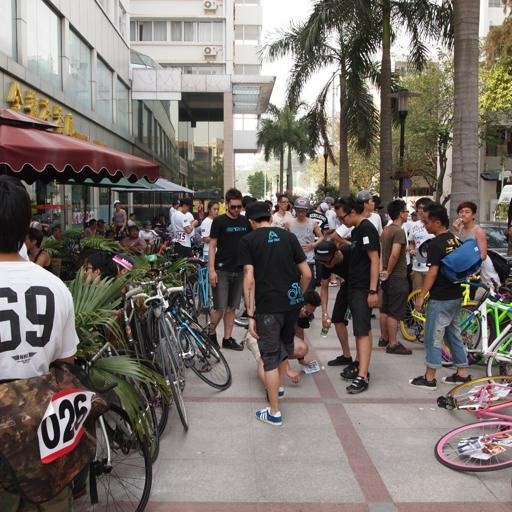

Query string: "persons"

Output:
[208,187,323,396]
[1,173,82,512]
[235,198,316,426]
[274,190,486,394]
[25,192,226,282]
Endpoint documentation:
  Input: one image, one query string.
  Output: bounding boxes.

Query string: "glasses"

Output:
[229,204,243,210]
[336,213,348,221]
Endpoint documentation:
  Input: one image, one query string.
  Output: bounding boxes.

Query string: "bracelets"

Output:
[246,312,257,321]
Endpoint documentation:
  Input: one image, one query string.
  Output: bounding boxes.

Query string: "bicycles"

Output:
[399,274,512,473]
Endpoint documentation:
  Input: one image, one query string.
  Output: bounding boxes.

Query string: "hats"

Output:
[246,202,271,220]
[314,240,336,263]
[180,198,195,207]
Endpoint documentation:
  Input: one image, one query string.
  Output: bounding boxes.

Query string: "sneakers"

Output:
[204,334,472,426]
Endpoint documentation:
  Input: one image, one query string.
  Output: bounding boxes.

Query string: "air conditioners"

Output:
[203,45,217,56]
[202,0,217,10]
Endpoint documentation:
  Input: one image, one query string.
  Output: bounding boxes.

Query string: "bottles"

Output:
[321,318,332,337]
[344,308,352,321]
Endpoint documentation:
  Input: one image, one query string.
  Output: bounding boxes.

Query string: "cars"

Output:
[479,221,511,284]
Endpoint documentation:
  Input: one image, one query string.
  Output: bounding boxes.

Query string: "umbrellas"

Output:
[1,107,161,186]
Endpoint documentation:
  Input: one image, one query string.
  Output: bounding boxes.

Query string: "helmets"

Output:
[293,190,372,212]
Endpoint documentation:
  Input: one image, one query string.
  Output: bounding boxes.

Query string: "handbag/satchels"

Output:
[440,239,483,282]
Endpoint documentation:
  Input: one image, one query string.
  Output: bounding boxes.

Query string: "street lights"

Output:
[276,174,281,194]
[284,168,291,192]
[386,88,421,198]
[319,141,333,196]
